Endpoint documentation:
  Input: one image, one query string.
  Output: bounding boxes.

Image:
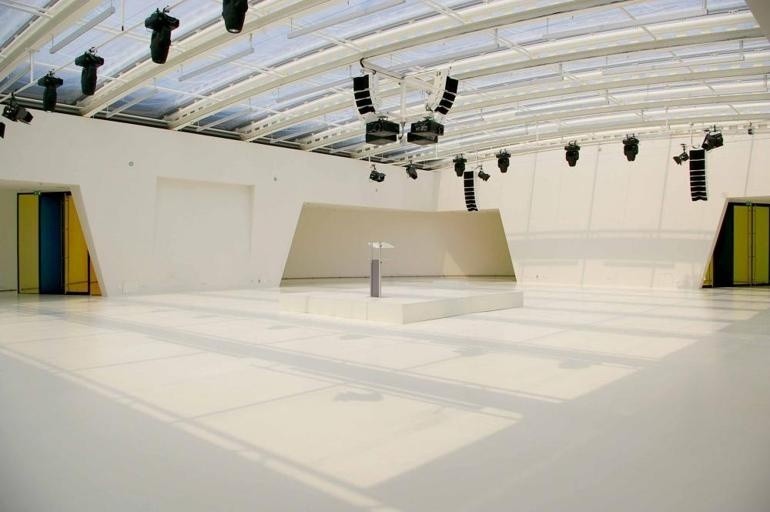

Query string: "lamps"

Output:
[495,152,512,173]
[478,171,491,181]
[564,141,580,167]
[408,122,443,142]
[0,1,251,142]
[675,152,688,164]
[370,171,384,181]
[702,130,723,150]
[407,166,418,180]
[366,119,398,143]
[622,135,638,160]
[453,157,468,177]
